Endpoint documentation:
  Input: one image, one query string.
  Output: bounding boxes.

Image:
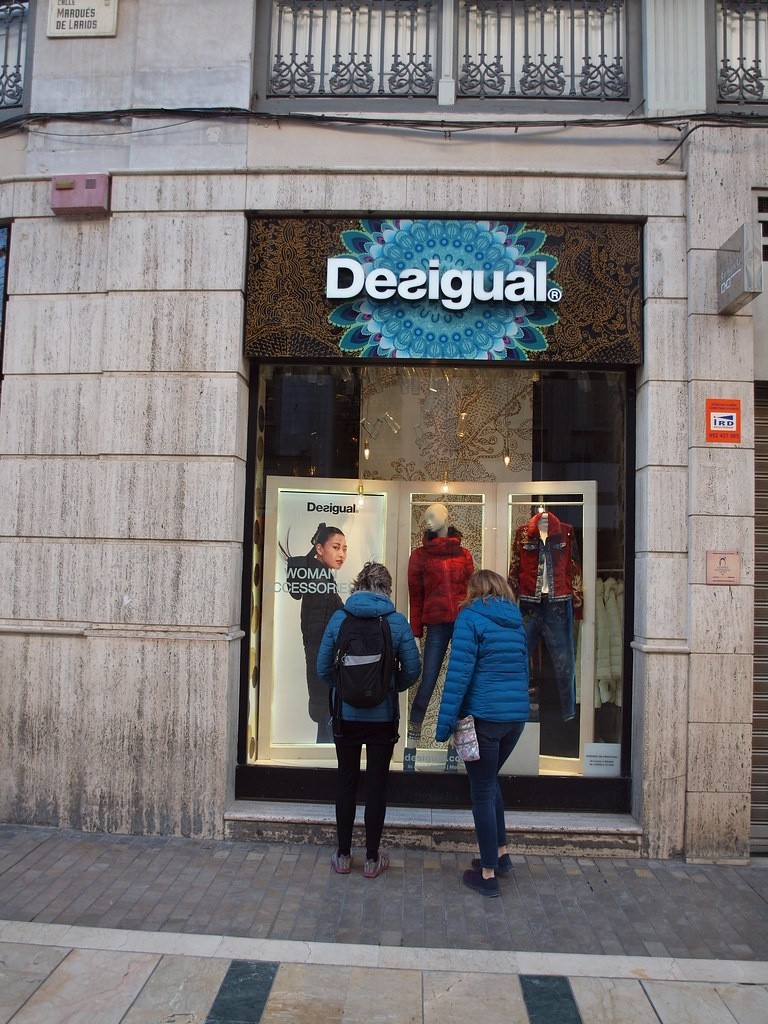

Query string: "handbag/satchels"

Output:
[453,715,480,761]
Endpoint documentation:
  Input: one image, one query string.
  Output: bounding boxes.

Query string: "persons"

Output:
[401,506,474,772]
[317,562,422,878]
[433,570,531,896]
[286,523,347,743]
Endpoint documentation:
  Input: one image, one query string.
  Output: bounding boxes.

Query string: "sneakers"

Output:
[463,870,500,898]
[331,848,353,874]
[471,853,515,876]
[362,851,389,878]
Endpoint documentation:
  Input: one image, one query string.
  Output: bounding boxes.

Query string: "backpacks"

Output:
[328,608,401,747]
